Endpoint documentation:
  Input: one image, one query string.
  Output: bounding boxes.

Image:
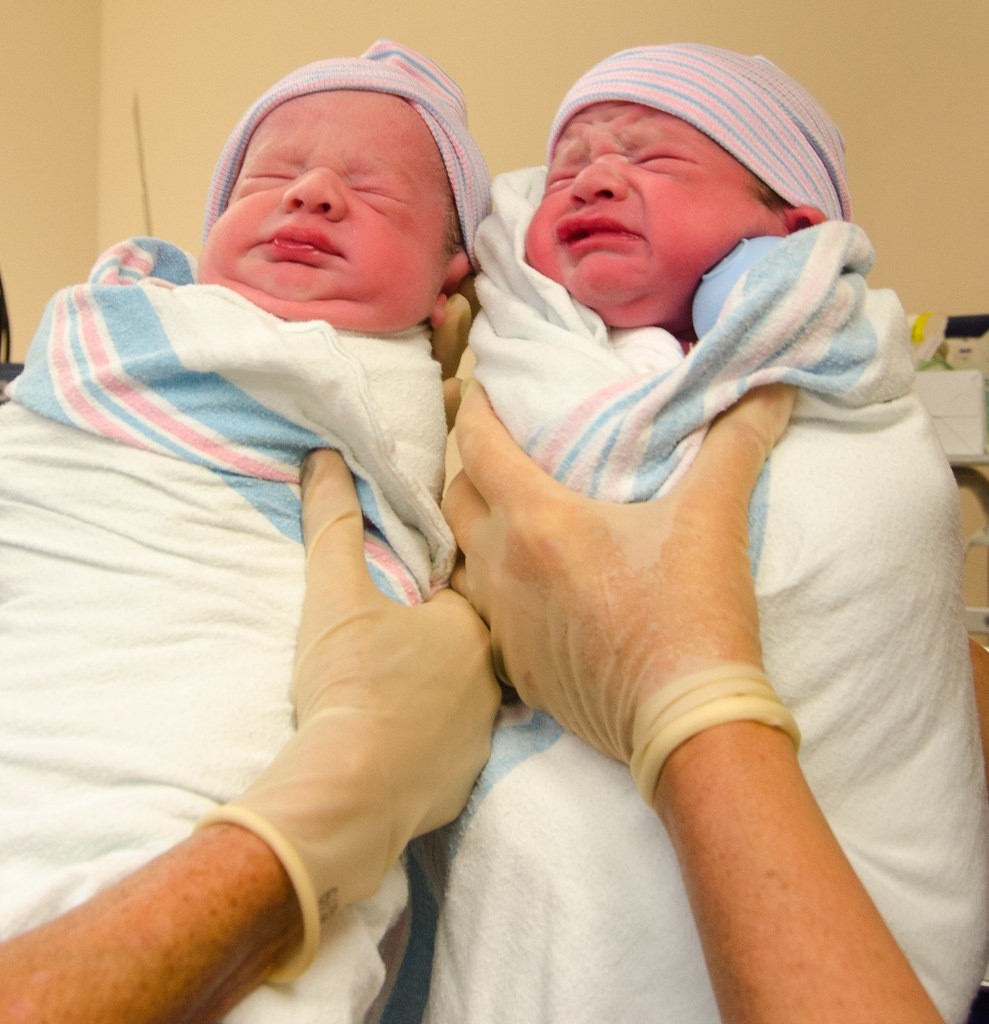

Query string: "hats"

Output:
[204,39,490,273]
[548,42,852,224]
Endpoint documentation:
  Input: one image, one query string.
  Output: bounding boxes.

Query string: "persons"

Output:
[0,35,522,1024]
[416,44,989,1024]
[0,297,989,1024]
